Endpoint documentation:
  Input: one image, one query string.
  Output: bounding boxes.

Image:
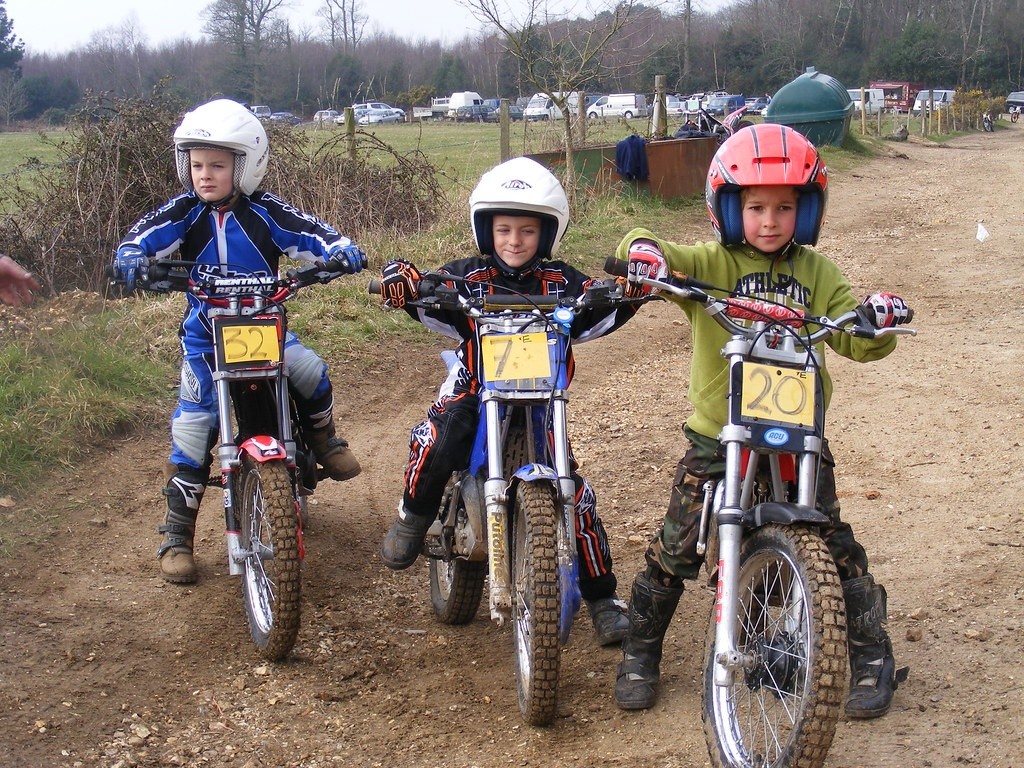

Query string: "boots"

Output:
[296,379,362,481]
[841,573,910,718]
[156,458,210,583]
[616,571,685,710]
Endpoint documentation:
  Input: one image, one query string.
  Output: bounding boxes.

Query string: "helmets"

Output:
[173,99,268,196]
[469,157,569,260]
[706,124,828,247]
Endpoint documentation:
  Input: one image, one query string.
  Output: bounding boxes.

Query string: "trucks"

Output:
[448,91,484,120]
[523,91,585,120]
[413,97,450,121]
[586,93,648,119]
[251,106,271,120]
[706,95,745,116]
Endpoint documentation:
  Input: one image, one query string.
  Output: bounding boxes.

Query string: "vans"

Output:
[912,90,956,118]
[846,89,885,114]
[456,105,499,122]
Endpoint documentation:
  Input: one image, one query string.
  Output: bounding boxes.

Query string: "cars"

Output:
[334,108,402,128]
[487,106,523,123]
[1005,92,1024,114]
[482,99,500,105]
[745,97,771,115]
[648,92,730,117]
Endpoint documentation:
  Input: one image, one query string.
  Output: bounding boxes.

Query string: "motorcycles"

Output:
[604,257,916,768]
[983,111,994,132]
[369,270,667,728]
[1011,105,1021,123]
[106,261,345,662]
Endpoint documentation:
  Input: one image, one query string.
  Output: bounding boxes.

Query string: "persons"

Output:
[377,157,648,646]
[113,99,370,583]
[614,124,910,720]
[0,254,42,309]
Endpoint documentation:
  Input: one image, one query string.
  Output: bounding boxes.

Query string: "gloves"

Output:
[863,294,913,328]
[627,239,669,298]
[328,244,368,274]
[614,274,649,308]
[380,260,422,308]
[113,244,151,291]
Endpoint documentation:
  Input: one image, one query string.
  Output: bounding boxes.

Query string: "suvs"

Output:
[352,103,405,117]
[270,112,301,125]
[312,110,339,122]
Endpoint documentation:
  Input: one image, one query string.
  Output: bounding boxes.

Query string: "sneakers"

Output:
[381,498,438,569]
[587,592,629,644]
